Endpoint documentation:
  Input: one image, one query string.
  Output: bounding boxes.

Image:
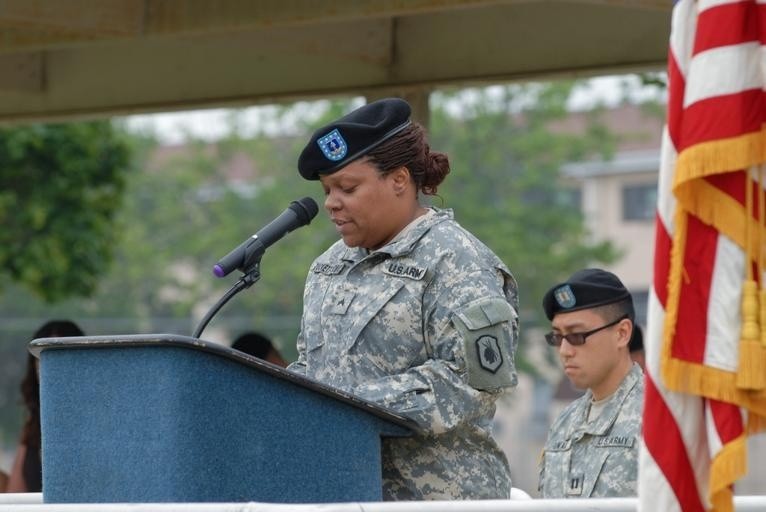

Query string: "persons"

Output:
[0,319,85,491]
[231,330,289,368]
[536,270,644,499]
[283,97,519,500]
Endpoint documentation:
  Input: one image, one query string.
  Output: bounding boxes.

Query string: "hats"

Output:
[298,97,412,181]
[542,268,630,322]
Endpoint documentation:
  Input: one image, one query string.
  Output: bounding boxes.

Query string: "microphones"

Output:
[213,197,318,278]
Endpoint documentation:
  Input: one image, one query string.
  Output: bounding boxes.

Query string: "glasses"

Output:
[544,314,628,347]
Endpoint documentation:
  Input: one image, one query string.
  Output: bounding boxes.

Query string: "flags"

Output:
[639,0,766,512]
